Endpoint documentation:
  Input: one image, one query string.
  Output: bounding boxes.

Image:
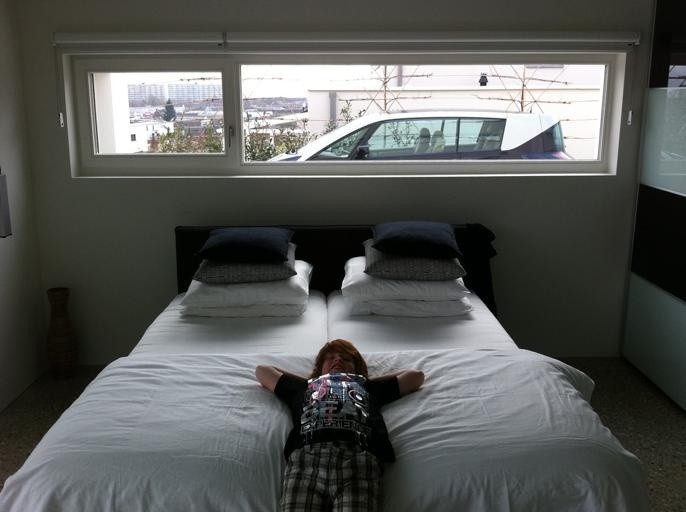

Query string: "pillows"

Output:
[177,228,313,315]
[341,220,473,316]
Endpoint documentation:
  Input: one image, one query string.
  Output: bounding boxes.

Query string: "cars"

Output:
[269,104,580,162]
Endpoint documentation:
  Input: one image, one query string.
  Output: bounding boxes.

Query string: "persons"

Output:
[255,339,424,511]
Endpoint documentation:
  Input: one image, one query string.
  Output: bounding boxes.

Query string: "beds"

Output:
[0,223,646,512]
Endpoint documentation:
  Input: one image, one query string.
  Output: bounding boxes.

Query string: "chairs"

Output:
[428,131,443,154]
[413,128,430,155]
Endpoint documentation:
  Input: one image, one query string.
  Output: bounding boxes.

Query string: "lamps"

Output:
[0,166,12,238]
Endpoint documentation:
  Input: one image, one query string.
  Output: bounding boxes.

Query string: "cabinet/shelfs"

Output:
[622,272,686,410]
[640,87,686,197]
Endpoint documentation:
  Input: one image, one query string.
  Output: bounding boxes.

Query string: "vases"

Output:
[45,287,80,379]
